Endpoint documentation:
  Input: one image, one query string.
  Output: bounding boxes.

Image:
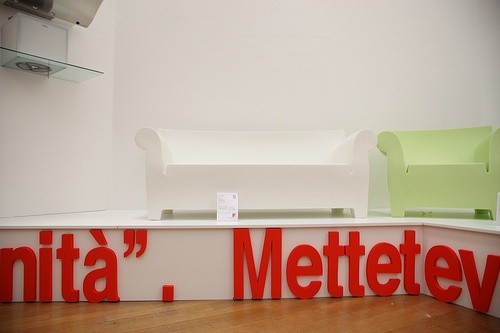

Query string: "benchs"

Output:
[135,127,378,221]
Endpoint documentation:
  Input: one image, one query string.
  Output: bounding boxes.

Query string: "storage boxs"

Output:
[1,12,70,77]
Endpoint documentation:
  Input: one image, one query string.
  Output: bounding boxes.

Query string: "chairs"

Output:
[376,127,498,220]
